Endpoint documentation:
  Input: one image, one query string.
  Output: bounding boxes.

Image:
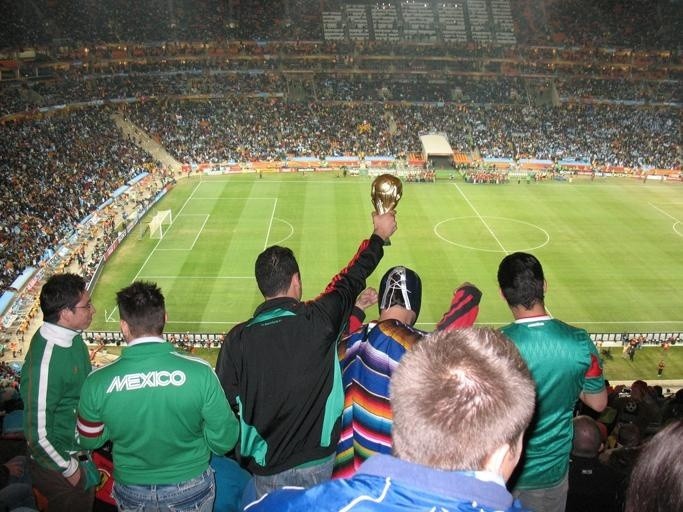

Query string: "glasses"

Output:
[71,302,91,310]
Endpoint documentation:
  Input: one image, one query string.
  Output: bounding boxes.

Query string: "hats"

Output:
[378,266,422,324]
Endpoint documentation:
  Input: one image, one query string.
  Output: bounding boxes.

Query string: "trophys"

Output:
[366,174,403,246]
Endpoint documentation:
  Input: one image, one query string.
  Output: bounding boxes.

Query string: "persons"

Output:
[248,326,537,511]
[193,0,682,189]
[0,0,193,252]
[335,266,483,482]
[2,267,115,510]
[565,332,679,508]
[76,280,241,509]
[213,209,398,498]
[493,252,608,510]
[493,252,608,512]
